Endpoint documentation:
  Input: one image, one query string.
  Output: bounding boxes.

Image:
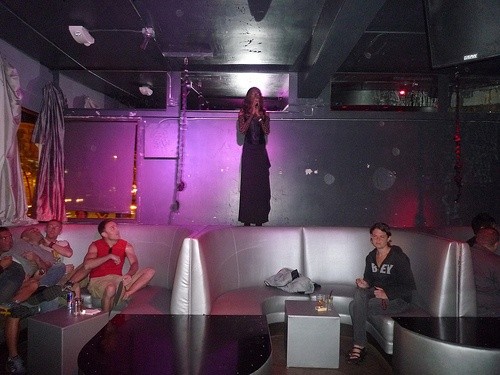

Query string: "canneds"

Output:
[66,291,75,310]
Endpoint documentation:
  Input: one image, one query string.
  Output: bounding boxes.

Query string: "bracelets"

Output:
[49,242,55,248]
[258,118,262,121]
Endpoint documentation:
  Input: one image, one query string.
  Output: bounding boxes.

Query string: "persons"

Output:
[83,220,156,317]
[237,87,271,227]
[8,228,66,320]
[466,214,497,249]
[344,222,416,366]
[38,220,80,304]
[467,226,500,318]
[0,226,38,375]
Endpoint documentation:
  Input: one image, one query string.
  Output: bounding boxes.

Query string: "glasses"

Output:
[371,234,388,239]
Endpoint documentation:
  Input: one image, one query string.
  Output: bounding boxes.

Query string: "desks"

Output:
[285,300,341,369]
[77,314,273,375]
[391,316,500,375]
[27,305,110,375]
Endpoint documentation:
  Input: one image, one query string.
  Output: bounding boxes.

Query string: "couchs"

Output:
[6,223,500,356]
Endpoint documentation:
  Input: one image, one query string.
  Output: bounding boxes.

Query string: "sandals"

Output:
[347,345,367,364]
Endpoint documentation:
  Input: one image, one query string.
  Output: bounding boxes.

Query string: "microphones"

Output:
[256,103,258,105]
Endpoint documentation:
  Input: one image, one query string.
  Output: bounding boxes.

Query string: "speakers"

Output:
[425,0,500,69]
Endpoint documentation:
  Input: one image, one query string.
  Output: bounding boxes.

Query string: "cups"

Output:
[324,296,333,311]
[72,298,84,316]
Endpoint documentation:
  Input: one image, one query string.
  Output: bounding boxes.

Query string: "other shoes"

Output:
[38,285,63,301]
[256,222,262,226]
[7,354,26,375]
[113,281,126,306]
[244,222,250,226]
[0,301,18,315]
